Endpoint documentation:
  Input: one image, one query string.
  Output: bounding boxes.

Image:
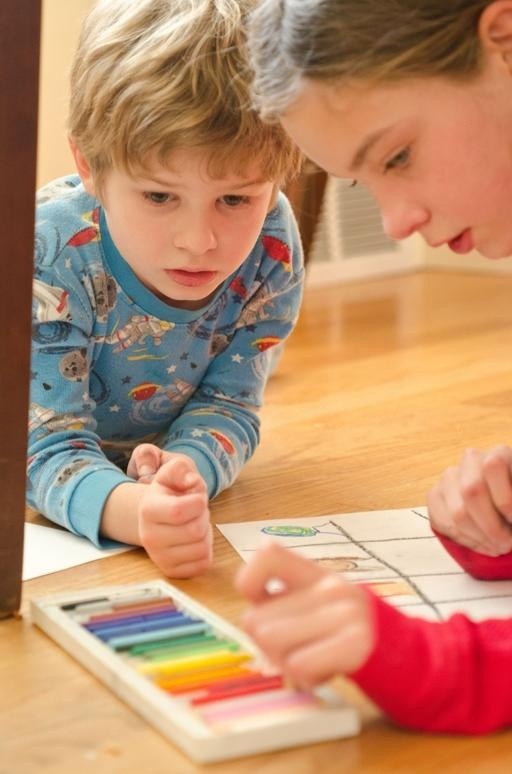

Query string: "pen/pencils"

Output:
[58,595,287,705]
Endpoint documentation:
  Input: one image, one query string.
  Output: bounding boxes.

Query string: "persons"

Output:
[24,0,307,579]
[236,0,512,735]
